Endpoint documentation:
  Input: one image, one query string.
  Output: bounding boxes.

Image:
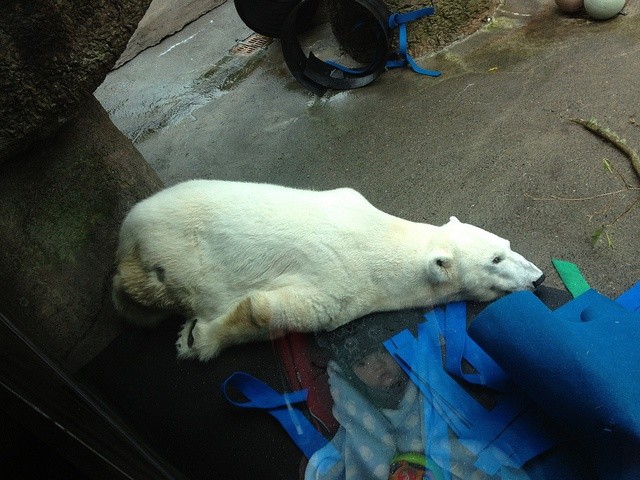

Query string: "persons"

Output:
[328,318,490,480]
